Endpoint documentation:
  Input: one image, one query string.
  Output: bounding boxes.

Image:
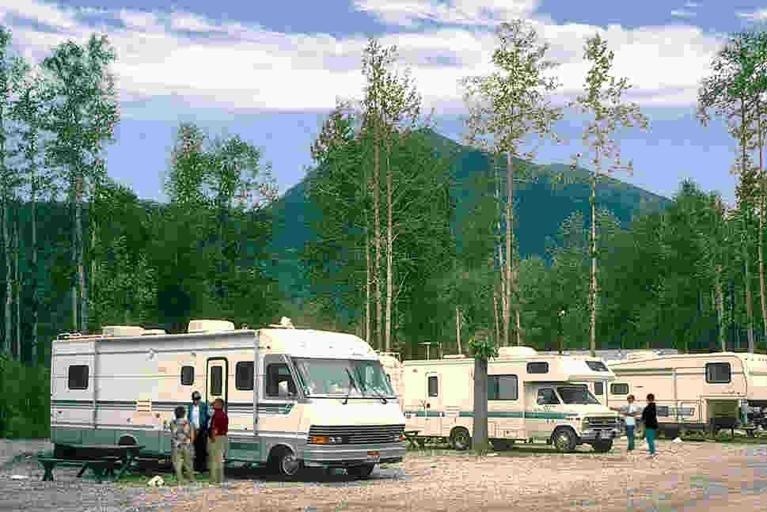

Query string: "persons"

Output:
[205,398,228,484]
[167,405,198,486]
[637,393,659,455]
[617,394,640,452]
[185,390,211,475]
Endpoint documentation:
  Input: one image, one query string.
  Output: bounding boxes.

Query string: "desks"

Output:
[403,430,420,449]
[51,441,142,481]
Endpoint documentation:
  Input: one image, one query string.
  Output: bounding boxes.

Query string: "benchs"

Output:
[37,456,158,484]
[408,437,425,449]
